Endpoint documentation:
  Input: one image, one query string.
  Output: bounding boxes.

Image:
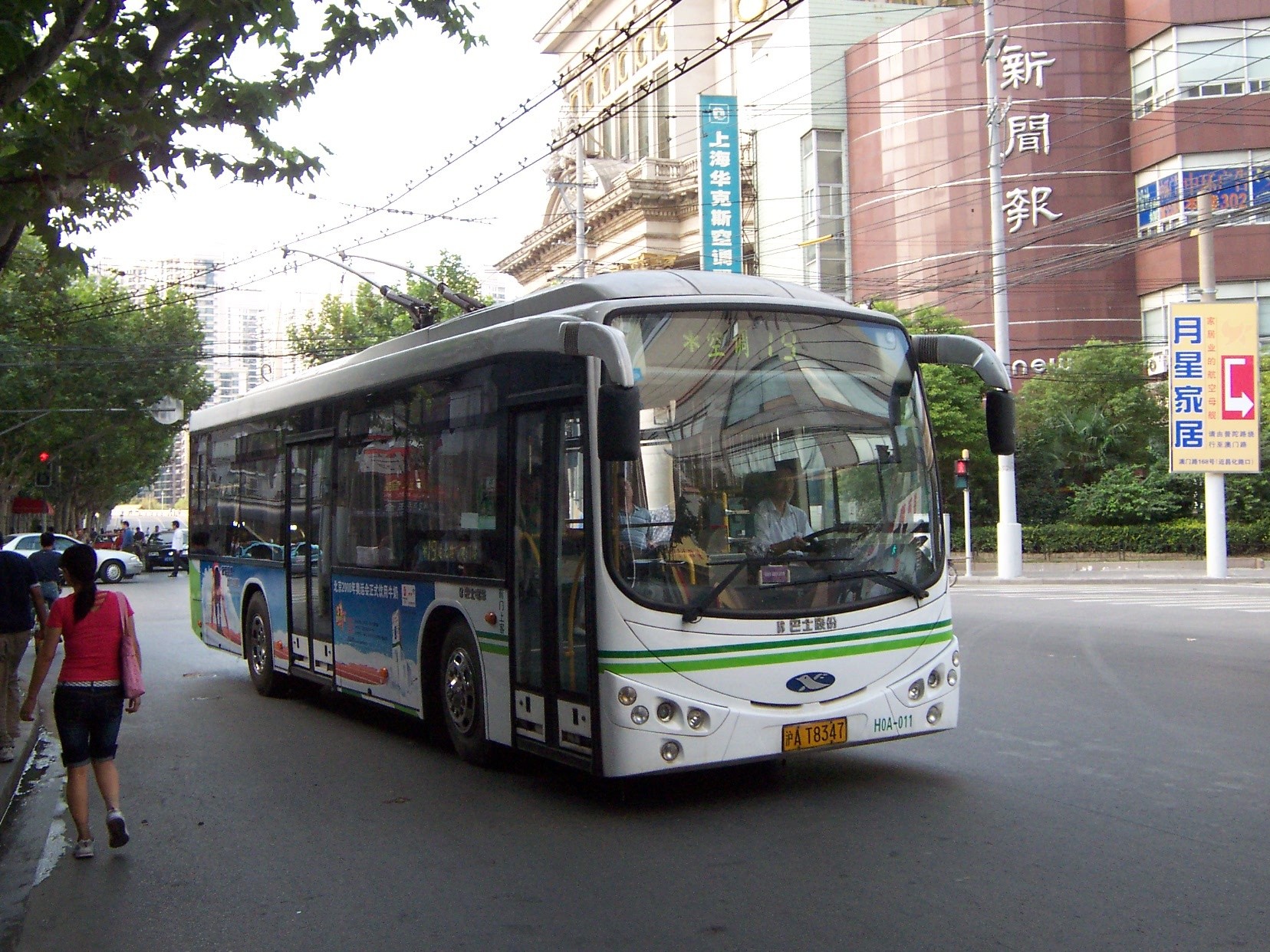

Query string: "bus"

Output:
[188,245,1017,783]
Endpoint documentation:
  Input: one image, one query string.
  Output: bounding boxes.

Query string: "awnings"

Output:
[11,496,55,515]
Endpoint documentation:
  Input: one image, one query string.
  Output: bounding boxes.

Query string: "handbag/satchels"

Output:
[116,591,145,699]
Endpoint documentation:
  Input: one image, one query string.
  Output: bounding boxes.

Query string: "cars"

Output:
[0,532,143,584]
[233,540,320,576]
[142,528,188,573]
[91,533,118,550]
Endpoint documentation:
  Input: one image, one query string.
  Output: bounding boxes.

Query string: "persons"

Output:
[495,462,552,595]
[753,462,819,556]
[46,521,55,532]
[602,473,659,552]
[36,520,43,532]
[168,520,190,577]
[20,544,142,858]
[26,532,63,642]
[0,531,48,762]
[66,521,159,579]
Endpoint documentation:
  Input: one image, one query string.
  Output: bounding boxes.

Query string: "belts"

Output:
[57,679,121,687]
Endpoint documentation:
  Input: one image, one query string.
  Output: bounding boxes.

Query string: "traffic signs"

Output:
[1166,301,1260,474]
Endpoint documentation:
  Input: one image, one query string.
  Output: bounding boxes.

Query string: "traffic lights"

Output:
[35,449,52,487]
[953,459,969,490]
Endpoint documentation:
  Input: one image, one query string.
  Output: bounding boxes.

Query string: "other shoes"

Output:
[0,746,14,761]
[74,835,95,857]
[106,808,130,848]
[168,572,174,577]
